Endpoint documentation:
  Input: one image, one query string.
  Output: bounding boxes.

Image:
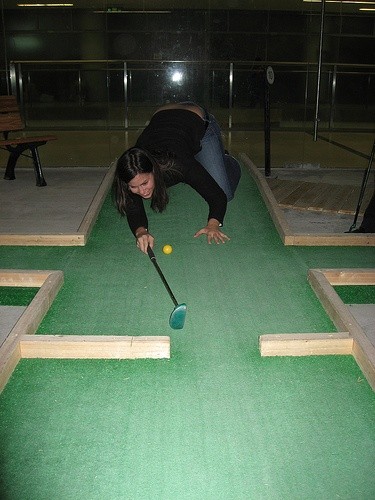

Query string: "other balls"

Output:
[163,245,173,255]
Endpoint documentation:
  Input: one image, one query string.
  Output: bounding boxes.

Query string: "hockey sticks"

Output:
[146,244,187,330]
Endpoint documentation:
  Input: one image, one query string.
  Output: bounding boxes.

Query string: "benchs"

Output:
[0,95,58,186]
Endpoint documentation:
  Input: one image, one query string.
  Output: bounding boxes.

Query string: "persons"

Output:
[113,103,244,255]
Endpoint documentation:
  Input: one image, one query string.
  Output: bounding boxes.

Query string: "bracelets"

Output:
[135,228,148,237]
